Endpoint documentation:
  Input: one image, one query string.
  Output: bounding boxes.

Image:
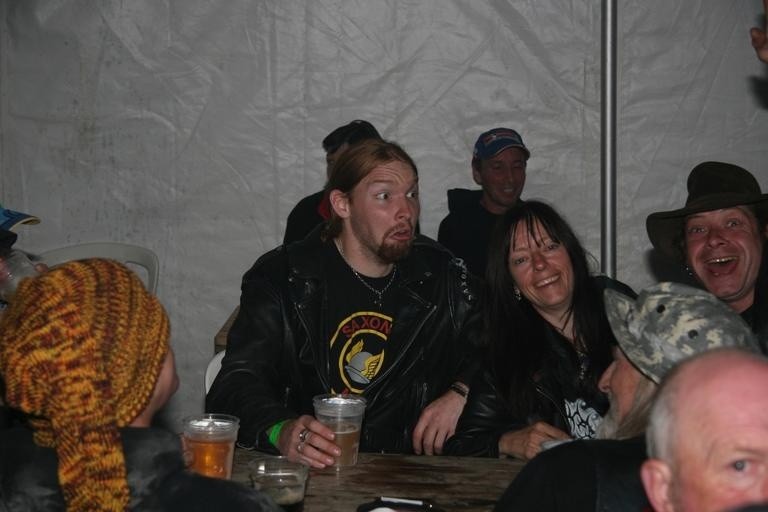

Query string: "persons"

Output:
[639,342,768,512]
[205,136,498,469]
[281,116,385,246]
[437,127,531,268]
[491,280,765,511]
[0,256,288,512]
[442,198,639,463]
[0,206,52,308]
[644,161,768,357]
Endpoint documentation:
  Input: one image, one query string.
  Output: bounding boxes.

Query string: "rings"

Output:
[295,441,306,454]
[297,428,311,440]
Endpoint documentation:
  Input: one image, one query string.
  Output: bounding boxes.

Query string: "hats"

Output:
[0,257,170,512]
[322,120,383,171]
[646,161,768,271]
[0,208,43,233]
[472,127,531,166]
[604,282,761,386]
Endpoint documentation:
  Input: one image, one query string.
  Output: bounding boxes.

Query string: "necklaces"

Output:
[552,307,575,337]
[335,234,400,305]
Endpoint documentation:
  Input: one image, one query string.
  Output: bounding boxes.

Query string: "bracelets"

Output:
[447,382,471,401]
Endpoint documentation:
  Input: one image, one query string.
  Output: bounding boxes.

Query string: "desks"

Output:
[230,447,530,512]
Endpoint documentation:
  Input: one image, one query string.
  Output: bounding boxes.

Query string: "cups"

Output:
[313,393,367,467]
[1,252,39,304]
[250,456,311,510]
[178,412,239,478]
